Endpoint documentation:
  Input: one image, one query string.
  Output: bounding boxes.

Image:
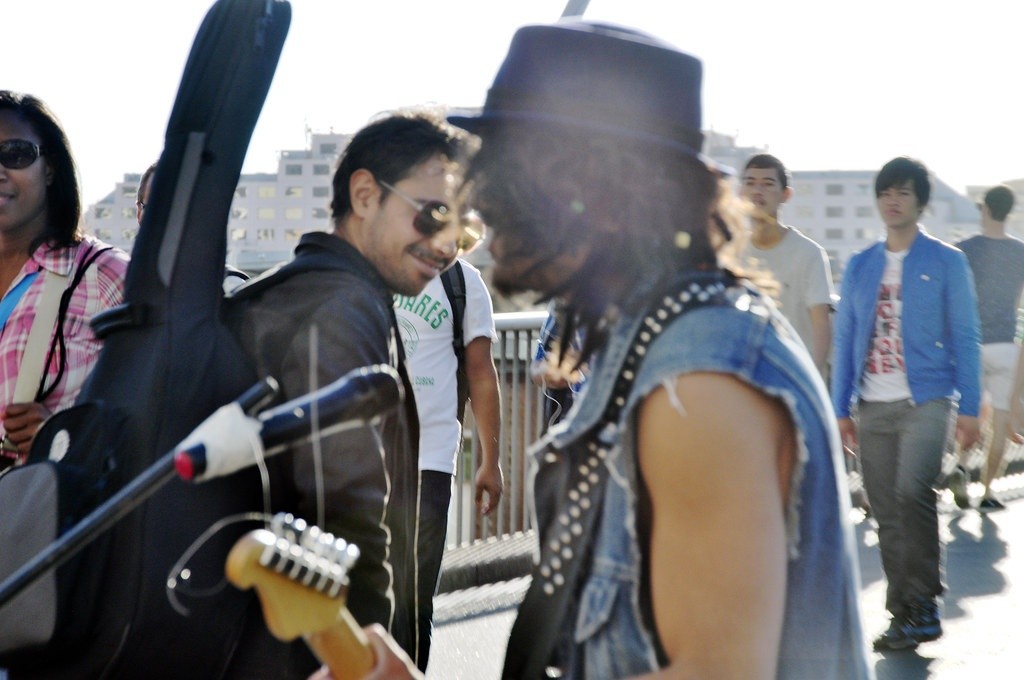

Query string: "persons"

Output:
[393,258,502,680]
[137,162,252,294]
[529,296,597,400]
[830,157,981,651]
[740,154,831,376]
[0,90,132,478]
[310,20,868,680]
[224,113,484,680]
[953,187,1024,509]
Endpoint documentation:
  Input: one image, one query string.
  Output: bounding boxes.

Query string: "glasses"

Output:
[0,138,46,169]
[378,178,485,251]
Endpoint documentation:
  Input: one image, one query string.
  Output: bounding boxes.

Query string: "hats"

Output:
[446,18,735,180]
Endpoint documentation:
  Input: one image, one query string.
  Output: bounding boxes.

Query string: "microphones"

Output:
[175,363,406,481]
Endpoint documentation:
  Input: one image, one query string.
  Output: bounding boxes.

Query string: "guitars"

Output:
[223,512,395,680]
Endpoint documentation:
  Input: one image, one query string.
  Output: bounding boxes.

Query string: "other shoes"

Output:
[948,467,970,509]
[873,599,942,649]
[980,499,1005,511]
